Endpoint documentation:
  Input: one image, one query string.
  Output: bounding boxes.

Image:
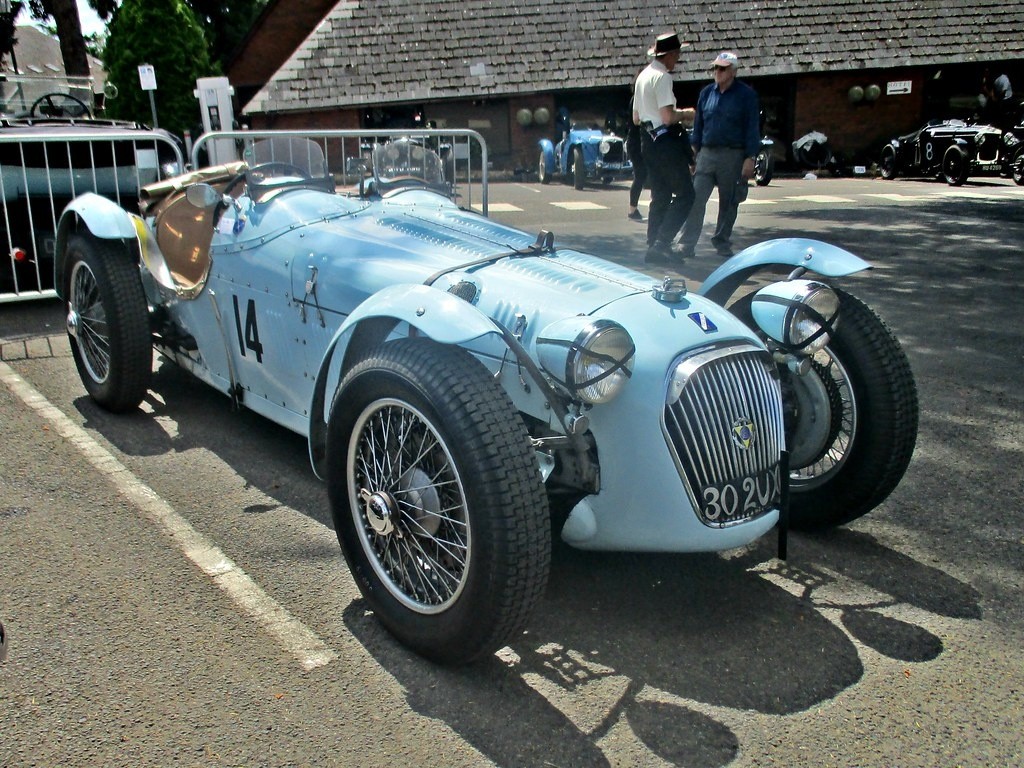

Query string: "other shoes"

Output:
[716,243,733,257]
[671,243,697,258]
[645,240,681,265]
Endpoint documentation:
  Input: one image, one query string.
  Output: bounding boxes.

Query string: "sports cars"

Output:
[534,125,635,192]
[879,120,1024,187]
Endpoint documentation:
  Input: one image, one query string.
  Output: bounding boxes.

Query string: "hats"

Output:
[712,51,740,67]
[647,30,691,56]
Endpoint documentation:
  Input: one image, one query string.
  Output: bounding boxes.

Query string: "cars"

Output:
[51,137,923,668]
[0,73,184,290]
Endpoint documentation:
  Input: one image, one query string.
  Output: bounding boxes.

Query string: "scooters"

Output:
[752,111,777,188]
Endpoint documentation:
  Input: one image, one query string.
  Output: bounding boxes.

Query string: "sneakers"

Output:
[628,209,649,223]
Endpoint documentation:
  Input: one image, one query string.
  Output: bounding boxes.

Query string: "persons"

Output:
[626,32,760,267]
[978,64,1013,131]
